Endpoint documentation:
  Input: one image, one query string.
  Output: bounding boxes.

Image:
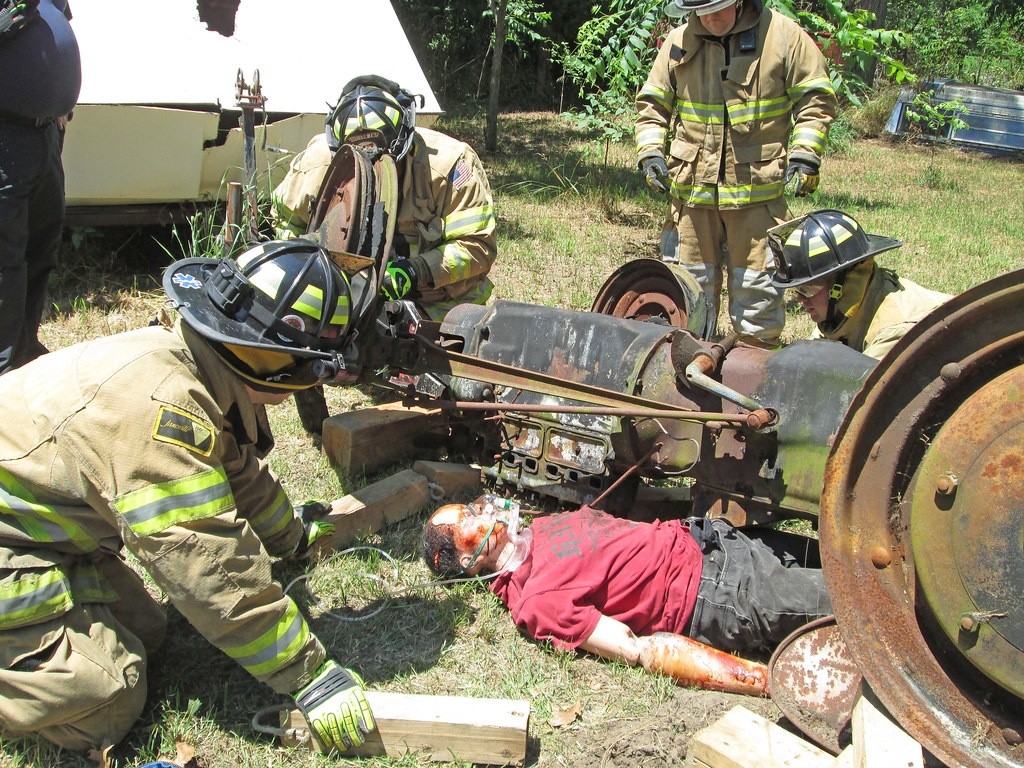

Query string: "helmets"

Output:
[664,0,737,18]
[162,238,354,359]
[326,75,415,162]
[771,210,903,288]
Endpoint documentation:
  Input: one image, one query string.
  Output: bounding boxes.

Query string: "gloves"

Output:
[288,658,376,751]
[640,156,668,193]
[282,500,336,560]
[783,159,820,197]
[379,259,417,300]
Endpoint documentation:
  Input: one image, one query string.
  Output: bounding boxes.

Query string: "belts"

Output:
[0,109,73,129]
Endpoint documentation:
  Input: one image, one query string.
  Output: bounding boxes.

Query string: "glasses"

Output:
[793,272,840,297]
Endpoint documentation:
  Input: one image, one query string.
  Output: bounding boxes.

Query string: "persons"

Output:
[769,210,956,359]
[635,0,837,349]
[425,502,833,697]
[271,76,496,385]
[0,0,72,373]
[0,240,376,756]
